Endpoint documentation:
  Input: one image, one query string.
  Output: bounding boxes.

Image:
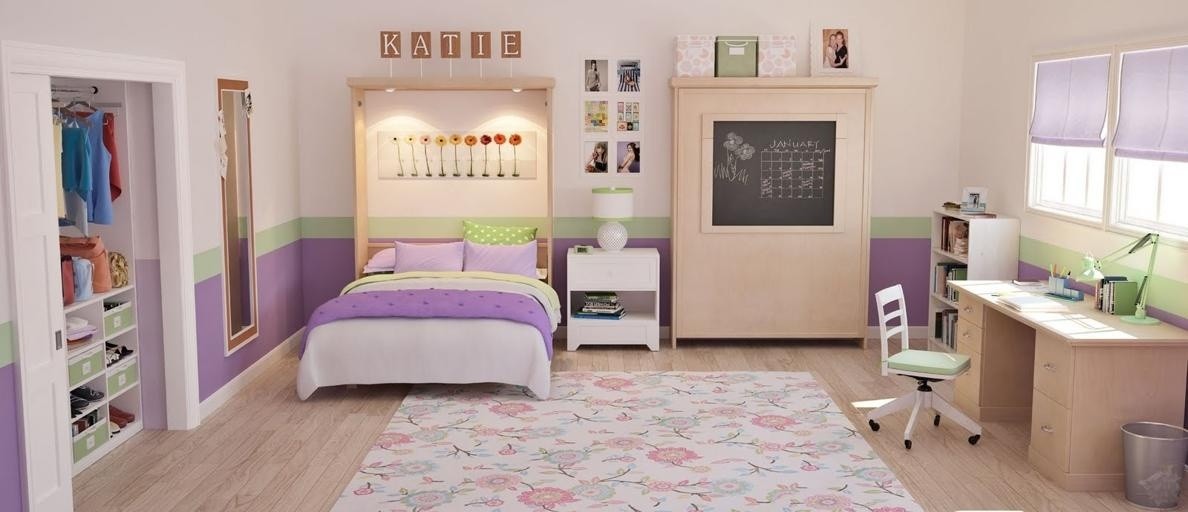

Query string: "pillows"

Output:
[362,248,395,274]
[393,239,465,273]
[463,239,537,279]
[462,219,538,245]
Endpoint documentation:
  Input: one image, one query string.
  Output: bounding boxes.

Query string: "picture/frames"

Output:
[580,54,644,178]
[961,186,986,212]
[701,113,848,233]
[215,78,261,357]
[809,21,861,76]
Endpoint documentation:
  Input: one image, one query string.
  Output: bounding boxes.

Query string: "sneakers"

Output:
[110,422,120,433]
[68,393,89,407]
[72,387,104,401]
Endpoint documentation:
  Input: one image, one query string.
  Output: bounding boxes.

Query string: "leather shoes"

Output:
[109,411,127,428]
[107,403,136,423]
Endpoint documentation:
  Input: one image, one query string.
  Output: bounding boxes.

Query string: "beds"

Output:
[296,77,560,402]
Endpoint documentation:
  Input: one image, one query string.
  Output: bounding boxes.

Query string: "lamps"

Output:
[592,188,633,251]
[1075,233,1160,324]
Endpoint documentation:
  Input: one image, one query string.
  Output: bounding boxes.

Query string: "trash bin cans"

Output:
[1120,422,1188,507]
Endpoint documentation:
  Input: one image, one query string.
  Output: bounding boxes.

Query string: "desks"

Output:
[945,279,1188,492]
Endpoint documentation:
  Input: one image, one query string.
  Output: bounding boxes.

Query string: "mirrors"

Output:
[223,90,247,333]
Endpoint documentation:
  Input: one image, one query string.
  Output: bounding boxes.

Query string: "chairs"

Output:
[867,284,983,449]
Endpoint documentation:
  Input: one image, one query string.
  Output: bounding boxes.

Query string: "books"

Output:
[932,263,964,302]
[940,218,970,254]
[576,291,627,320]
[933,309,960,349]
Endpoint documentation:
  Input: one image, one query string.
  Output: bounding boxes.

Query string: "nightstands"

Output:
[567,247,660,352]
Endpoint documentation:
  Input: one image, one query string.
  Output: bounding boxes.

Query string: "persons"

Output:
[584,142,609,172]
[618,141,637,172]
[588,60,600,92]
[826,31,847,69]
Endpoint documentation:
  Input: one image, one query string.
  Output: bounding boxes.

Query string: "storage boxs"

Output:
[70,417,116,461]
[67,346,106,385]
[103,301,132,335]
[108,359,139,395]
[758,34,799,76]
[716,36,757,77]
[672,34,716,77]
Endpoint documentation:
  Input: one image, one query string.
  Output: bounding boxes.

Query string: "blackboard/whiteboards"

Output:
[712,120,837,228]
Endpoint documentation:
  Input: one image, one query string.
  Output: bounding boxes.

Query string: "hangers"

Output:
[52,83,107,123]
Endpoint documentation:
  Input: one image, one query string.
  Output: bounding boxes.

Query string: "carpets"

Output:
[329,372,922,512]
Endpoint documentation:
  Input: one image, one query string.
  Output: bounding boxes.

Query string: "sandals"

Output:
[106,350,119,366]
[107,334,133,356]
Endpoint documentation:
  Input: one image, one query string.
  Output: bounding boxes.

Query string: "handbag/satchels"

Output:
[61,256,76,306]
[61,234,112,291]
[72,257,95,301]
[110,249,129,288]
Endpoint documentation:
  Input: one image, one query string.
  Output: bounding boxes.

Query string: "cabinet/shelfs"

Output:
[669,77,878,349]
[928,207,1020,353]
[51,75,143,478]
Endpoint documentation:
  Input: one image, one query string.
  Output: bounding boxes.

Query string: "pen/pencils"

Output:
[1066,272,1070,280]
[941,352,958,363]
[1050,263,1057,278]
[1059,266,1066,277]
